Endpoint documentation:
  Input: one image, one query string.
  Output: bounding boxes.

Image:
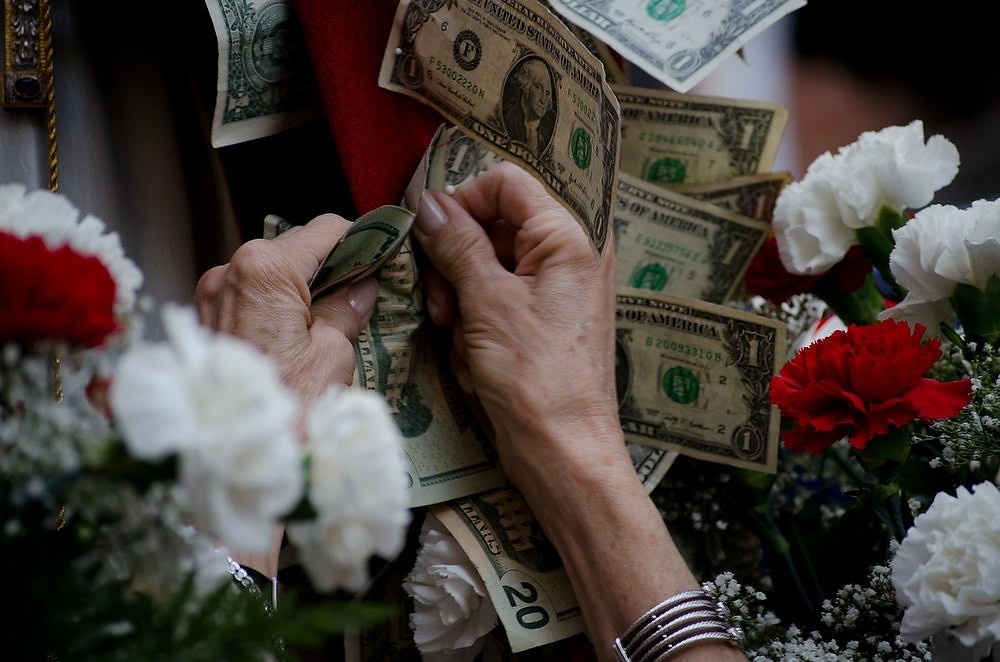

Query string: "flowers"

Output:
[686,118,1000,660]
[2,180,499,662]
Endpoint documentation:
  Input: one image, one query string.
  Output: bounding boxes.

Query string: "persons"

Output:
[124,159,762,662]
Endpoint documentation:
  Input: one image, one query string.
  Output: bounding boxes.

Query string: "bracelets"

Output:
[164,507,297,660]
[607,591,742,661]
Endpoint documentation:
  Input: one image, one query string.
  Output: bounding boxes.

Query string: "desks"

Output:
[294,0,1000,320]
